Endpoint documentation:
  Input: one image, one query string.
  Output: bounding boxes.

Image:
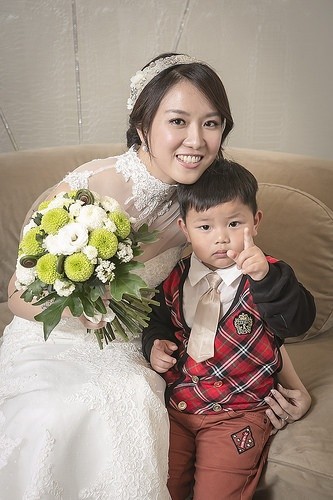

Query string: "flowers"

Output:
[8,189,161,350]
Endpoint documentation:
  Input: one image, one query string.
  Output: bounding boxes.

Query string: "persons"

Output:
[0,54,311,500]
[142,157,317,500]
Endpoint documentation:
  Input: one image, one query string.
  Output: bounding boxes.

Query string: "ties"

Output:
[186,272,223,363]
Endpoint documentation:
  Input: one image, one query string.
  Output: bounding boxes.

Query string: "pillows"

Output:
[231,182,333,343]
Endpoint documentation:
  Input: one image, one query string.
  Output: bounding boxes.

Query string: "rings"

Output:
[282,415,289,421]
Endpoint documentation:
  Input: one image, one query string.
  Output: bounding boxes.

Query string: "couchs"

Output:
[0,147,333,500]
